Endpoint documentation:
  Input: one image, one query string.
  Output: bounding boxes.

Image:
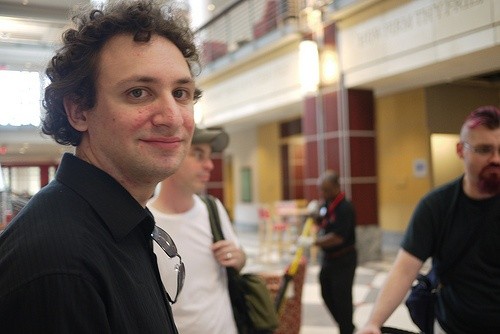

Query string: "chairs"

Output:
[264,254,308,334]
[257,198,321,268]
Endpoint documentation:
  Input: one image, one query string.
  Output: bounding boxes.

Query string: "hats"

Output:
[192,125,228,151]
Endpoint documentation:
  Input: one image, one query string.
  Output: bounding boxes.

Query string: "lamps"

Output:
[321,48,342,91]
[207,2,216,13]
[296,35,319,102]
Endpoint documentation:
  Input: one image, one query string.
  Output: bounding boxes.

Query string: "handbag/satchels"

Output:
[199,194,280,333]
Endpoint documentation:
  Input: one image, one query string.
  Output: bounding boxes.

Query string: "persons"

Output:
[298,170,357,334]
[145,125,246,334]
[357,106,500,334]
[0,0,203,334]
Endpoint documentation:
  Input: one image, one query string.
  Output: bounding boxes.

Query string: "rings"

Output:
[227,252,232,259]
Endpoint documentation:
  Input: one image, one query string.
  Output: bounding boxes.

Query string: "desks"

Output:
[236,39,249,49]
[277,205,315,255]
[254,269,284,291]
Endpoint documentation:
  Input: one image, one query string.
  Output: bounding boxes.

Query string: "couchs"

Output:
[249,1,280,40]
[200,35,229,65]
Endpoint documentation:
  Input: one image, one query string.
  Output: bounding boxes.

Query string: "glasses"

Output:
[151,225,184,303]
